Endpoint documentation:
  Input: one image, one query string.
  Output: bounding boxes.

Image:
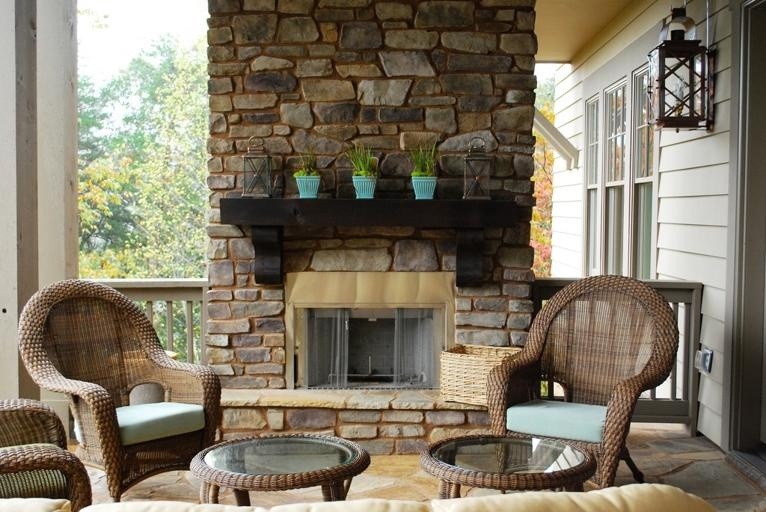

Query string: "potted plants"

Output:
[345,143,377,199]
[406,136,437,200]
[294,147,321,198]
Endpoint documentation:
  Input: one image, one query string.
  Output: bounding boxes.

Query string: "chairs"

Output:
[17,279,221,502]
[2,399,91,511]
[486,275,680,491]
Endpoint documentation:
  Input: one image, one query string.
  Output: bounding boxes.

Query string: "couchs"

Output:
[0,485,710,511]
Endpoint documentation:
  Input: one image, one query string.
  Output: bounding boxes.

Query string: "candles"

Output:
[676,85,684,115]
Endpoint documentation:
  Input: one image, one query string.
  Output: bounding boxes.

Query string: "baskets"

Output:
[439,343,523,410]
